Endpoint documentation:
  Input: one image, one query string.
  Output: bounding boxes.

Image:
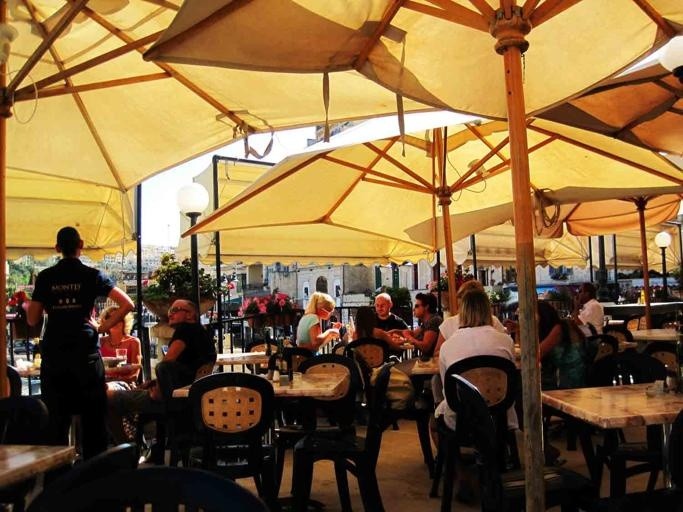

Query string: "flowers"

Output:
[131,250,236,303]
[426,267,475,291]
[238,287,299,328]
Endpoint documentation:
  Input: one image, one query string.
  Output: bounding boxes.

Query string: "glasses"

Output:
[170,306,190,314]
[321,308,335,317]
[415,304,419,309]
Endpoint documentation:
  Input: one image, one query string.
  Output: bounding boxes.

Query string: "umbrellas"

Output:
[533,193,683,328]
[531,59,683,156]
[146,0,683,512]
[181,112,683,317]
[470,220,593,282]
[0,167,142,385]
[199,223,441,372]
[0,0,447,397]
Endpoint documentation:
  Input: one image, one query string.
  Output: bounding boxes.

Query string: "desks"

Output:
[631,329,683,357]
[410,344,541,465]
[603,302,683,330]
[216,352,285,372]
[172,373,349,498]
[16,354,141,394]
[540,383,683,498]
[0,446,76,512]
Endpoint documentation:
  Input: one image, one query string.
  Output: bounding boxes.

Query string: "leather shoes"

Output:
[455,487,477,507]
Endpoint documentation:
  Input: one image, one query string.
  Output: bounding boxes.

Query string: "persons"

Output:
[435,281,509,357]
[393,293,444,377]
[373,293,409,331]
[98,307,142,399]
[28,227,134,461]
[432,289,518,503]
[296,292,342,361]
[572,282,604,337]
[347,306,401,350]
[502,300,589,390]
[620,283,683,304]
[117,299,215,425]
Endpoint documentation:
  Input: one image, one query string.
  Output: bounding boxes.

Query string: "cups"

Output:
[115,348,127,365]
[293,372,302,388]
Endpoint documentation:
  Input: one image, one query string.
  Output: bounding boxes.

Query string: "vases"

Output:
[246,312,290,329]
[431,291,462,311]
[432,291,458,311]
[146,298,217,324]
[150,325,175,361]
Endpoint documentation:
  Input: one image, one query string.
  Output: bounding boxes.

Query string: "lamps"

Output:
[655,232,672,298]
[177,183,209,325]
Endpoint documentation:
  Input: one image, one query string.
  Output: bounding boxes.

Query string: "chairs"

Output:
[579,321,633,370]
[441,373,593,512]
[642,341,683,376]
[577,351,673,495]
[6,366,21,398]
[23,442,268,512]
[245,340,280,375]
[266,362,395,512]
[120,361,214,468]
[0,396,48,512]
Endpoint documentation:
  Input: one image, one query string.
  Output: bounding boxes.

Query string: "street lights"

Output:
[652,232,673,305]
